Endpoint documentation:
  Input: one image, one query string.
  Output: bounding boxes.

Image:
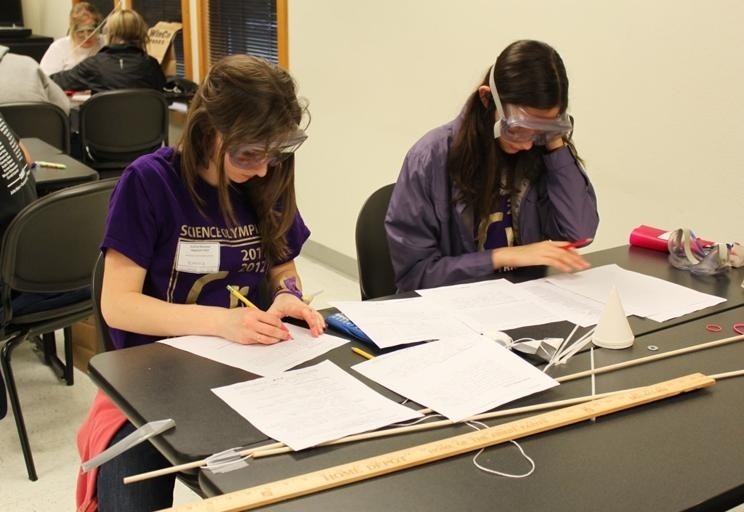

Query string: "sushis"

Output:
[326,313,374,344]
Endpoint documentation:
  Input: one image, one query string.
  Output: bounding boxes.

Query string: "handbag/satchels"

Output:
[271,276,304,301]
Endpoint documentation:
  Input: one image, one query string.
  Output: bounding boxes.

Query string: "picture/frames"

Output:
[198,305,744,512]
[88,245,744,475]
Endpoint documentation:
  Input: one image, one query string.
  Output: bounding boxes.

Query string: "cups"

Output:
[225,130,308,170]
[74,24,97,38]
[503,116,571,143]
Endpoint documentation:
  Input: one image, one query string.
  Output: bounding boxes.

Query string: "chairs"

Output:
[356,187,396,300]
[1,87,167,171]
[3,175,123,478]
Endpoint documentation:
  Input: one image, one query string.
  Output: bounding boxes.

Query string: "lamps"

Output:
[356,187,396,300]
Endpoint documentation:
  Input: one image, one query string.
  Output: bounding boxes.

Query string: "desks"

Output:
[198,305,744,512]
[88,245,744,475]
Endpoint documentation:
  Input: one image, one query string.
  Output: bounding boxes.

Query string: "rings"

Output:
[352,347,375,360]
[34,160,66,169]
[226,285,294,340]
[559,238,593,250]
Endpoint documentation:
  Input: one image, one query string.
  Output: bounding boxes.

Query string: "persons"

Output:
[49,9,168,167]
[75,54,327,512]
[0,112,38,301]
[0,44,72,117]
[383,40,599,292]
[39,2,107,77]
